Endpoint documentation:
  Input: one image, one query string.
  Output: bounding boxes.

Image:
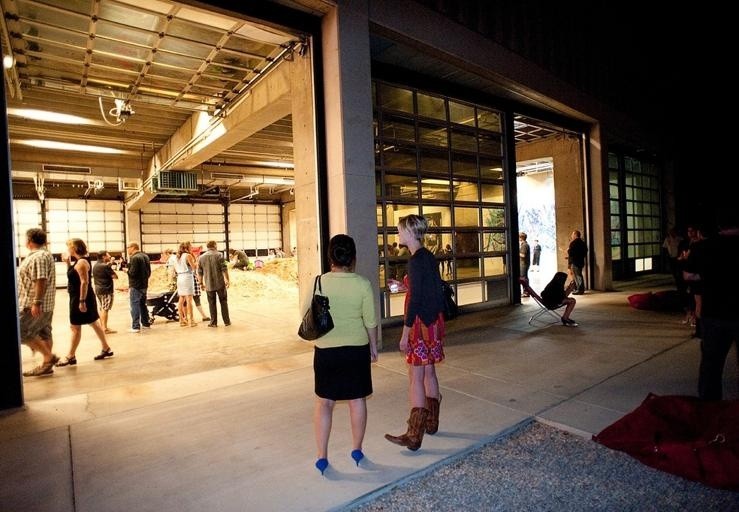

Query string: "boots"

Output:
[424,393,442,435]
[385,406,429,451]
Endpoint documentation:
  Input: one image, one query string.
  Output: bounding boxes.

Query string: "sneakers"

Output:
[104,327,118,334]
[23,354,60,378]
[128,325,150,333]
[180,317,232,327]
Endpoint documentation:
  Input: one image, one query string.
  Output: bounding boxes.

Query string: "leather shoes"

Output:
[572,291,584,295]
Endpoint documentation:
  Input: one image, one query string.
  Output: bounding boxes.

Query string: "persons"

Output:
[16,230,61,377]
[519,233,531,297]
[530,240,541,273]
[95,250,120,334]
[57,235,114,366]
[657,222,704,326]
[315,234,378,474]
[385,214,445,453]
[564,232,573,290]
[445,244,453,277]
[436,242,445,275]
[568,230,589,296]
[378,241,409,280]
[540,272,580,327]
[110,255,129,271]
[162,240,252,326]
[680,203,739,401]
[123,241,151,331]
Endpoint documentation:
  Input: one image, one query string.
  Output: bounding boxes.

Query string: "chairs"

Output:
[519,277,563,325]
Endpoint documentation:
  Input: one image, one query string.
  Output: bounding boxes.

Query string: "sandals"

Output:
[94,347,114,360]
[55,355,77,367]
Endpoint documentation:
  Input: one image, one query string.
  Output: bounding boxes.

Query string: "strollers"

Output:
[146,289,179,324]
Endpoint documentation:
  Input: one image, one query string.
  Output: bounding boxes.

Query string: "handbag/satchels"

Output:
[442,280,459,322]
[297,275,335,341]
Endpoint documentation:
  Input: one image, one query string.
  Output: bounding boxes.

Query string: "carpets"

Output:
[327,412,739,512]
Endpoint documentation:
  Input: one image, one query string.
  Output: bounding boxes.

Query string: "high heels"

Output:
[561,316,575,325]
[315,458,329,475]
[351,449,364,467]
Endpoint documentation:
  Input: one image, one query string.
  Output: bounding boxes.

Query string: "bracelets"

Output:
[79,298,87,303]
[32,300,43,306]
